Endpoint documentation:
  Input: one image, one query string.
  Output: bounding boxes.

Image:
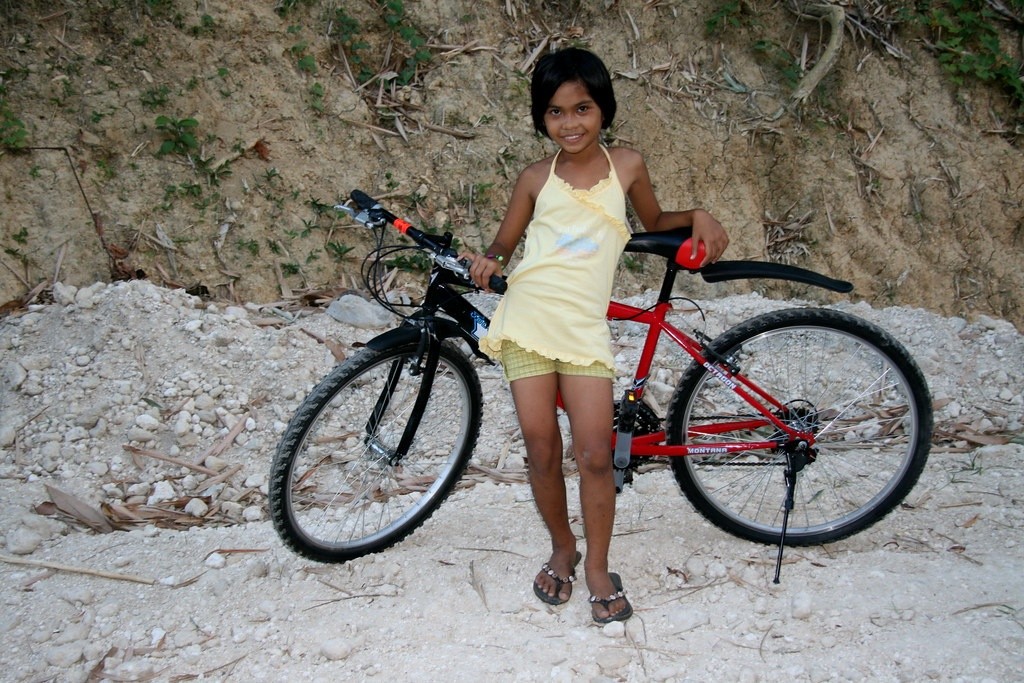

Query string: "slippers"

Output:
[589,572,633,623]
[533,551,582,605]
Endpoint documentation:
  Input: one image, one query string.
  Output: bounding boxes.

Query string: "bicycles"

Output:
[266,189,934,584]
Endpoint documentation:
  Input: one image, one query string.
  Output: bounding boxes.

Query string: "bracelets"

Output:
[484,253,505,270]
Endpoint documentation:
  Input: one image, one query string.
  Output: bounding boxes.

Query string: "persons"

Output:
[470,47,730,623]
